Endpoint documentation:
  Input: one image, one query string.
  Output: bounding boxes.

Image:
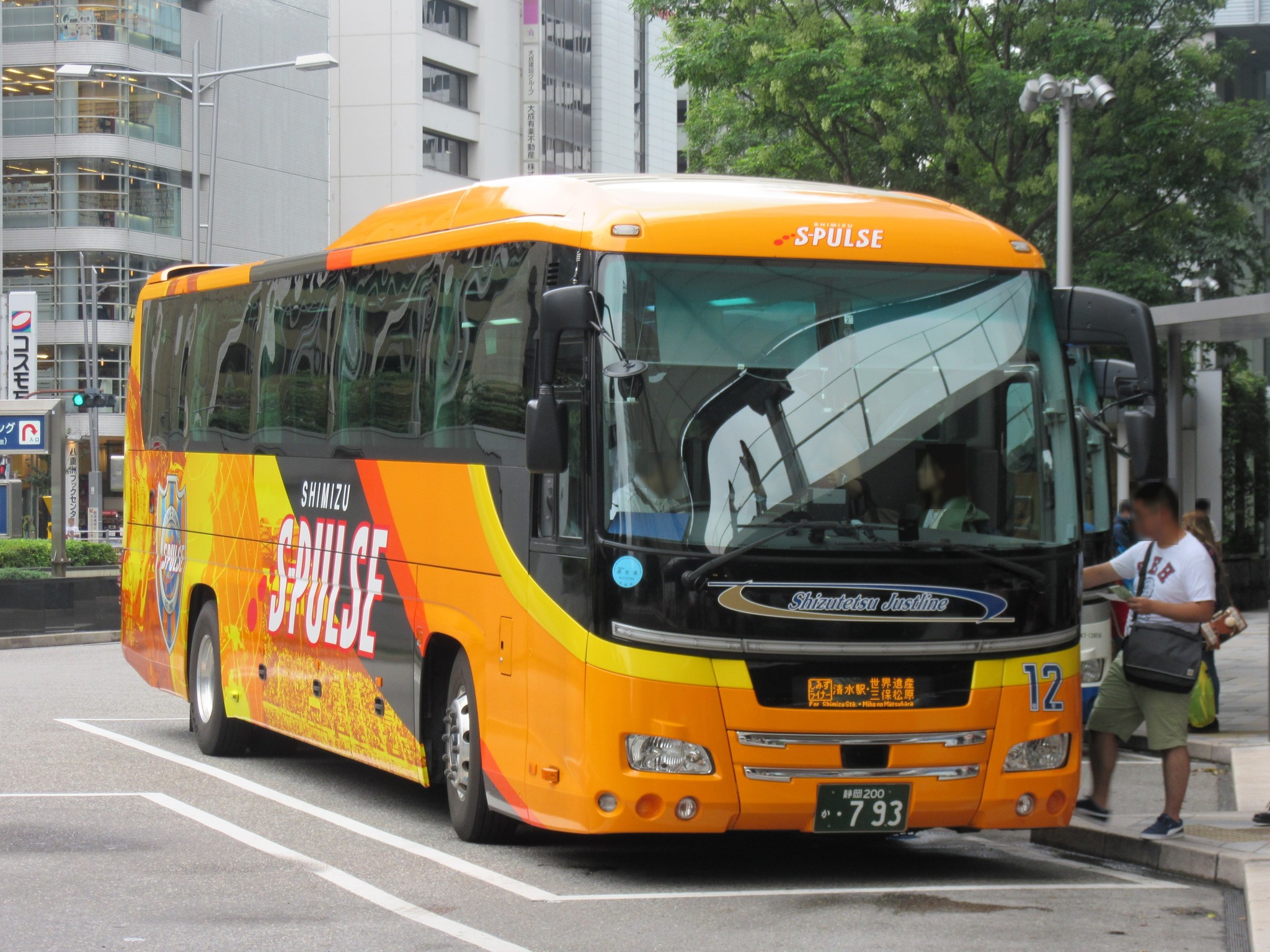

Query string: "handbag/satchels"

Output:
[1121,620,1207,694]
[1200,605,1248,653]
[1188,659,1213,727]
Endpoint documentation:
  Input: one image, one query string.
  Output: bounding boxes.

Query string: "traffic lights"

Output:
[0,465,6,480]
[72,393,117,408]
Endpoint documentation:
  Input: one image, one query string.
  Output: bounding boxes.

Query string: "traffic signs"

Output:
[1,410,51,455]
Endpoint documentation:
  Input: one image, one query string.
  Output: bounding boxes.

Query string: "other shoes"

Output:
[1187,717,1220,734]
[1252,812,1270,824]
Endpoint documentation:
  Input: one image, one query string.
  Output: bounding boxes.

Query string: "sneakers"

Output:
[1073,795,1112,825]
[1139,812,1184,839]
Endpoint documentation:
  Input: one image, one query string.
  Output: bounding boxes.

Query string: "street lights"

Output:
[54,13,340,265]
[1017,71,1118,286]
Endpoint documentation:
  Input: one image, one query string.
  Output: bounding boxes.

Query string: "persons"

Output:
[810,441,864,521]
[1180,498,1218,563]
[912,447,991,534]
[1177,512,1221,736]
[1112,497,1139,598]
[1076,477,1218,842]
[607,437,688,525]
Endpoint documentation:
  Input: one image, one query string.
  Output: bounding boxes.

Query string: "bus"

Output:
[1066,333,1157,727]
[118,170,1169,854]
